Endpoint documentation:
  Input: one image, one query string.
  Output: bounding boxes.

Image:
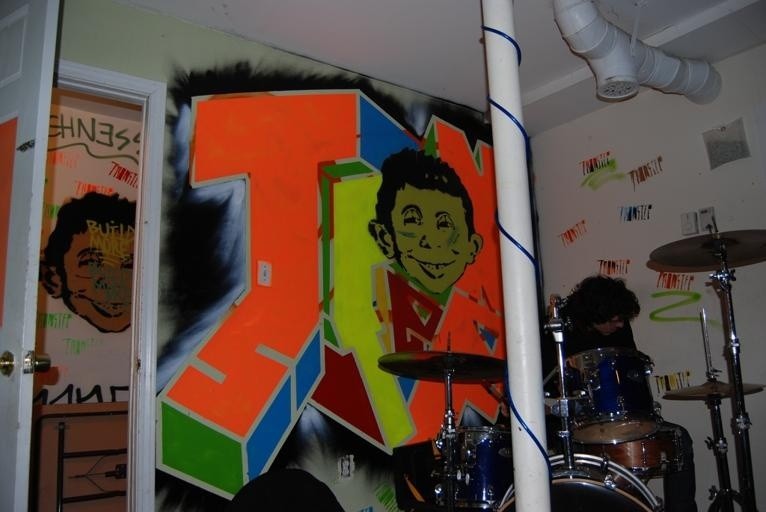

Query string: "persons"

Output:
[38,191,136,334]
[367,144,485,296]
[498,274,699,511]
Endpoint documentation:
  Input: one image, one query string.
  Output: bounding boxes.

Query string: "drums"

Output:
[433,424,514,510]
[592,430,690,475]
[544,347,663,446]
[496,453,659,512]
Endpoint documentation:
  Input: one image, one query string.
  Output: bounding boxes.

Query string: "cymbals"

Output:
[662,379,764,402]
[649,229,764,271]
[378,351,507,385]
